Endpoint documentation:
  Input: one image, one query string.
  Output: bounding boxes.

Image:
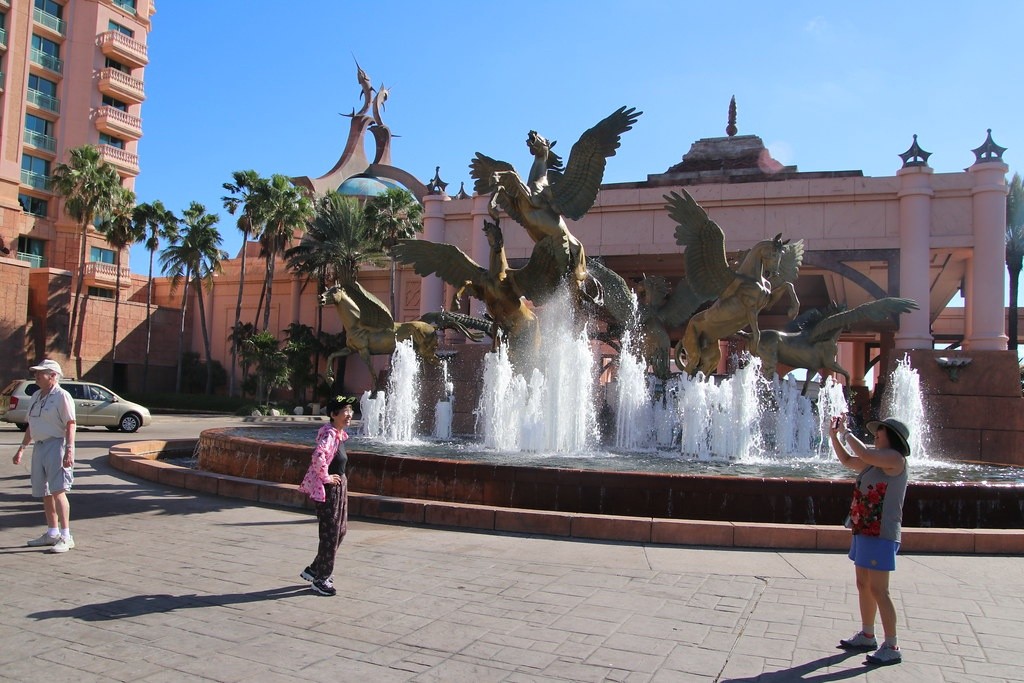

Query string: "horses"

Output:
[317,279,485,399]
[451,219,540,383]
[487,130,605,313]
[682,233,800,383]
[735,296,853,400]
[585,252,671,378]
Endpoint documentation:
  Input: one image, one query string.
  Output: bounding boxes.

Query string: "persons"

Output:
[12,358,77,553]
[828,414,911,666]
[299,395,359,596]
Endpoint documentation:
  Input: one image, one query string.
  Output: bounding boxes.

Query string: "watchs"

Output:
[65,444,74,448]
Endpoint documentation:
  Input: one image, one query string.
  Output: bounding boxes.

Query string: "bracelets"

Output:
[19,444,28,449]
[843,428,852,437]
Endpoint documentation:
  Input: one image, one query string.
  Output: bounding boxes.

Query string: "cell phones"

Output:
[836,417,841,429]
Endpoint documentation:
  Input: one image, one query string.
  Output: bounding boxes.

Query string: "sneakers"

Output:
[311,575,338,597]
[866,641,903,667]
[49,534,76,552]
[28,533,61,547]
[840,631,877,649]
[300,565,335,583]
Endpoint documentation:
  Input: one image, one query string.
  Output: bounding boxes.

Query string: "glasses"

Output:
[876,426,884,432]
[28,401,45,418]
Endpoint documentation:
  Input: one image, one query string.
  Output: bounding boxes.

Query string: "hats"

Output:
[321,393,357,413]
[868,417,911,456]
[29,360,63,378]
